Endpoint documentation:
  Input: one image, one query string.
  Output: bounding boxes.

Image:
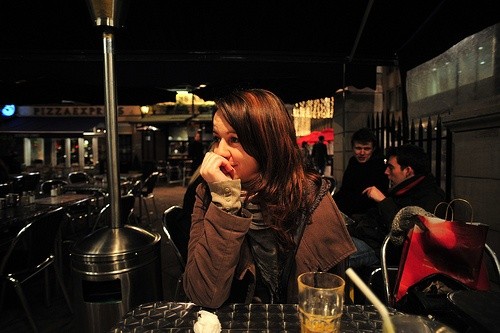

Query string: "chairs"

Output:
[0,168,158,333]
[162,205,190,300]
[380,231,500,306]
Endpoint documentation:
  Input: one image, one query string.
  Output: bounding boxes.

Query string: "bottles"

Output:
[51,184,61,196]
[22,191,35,204]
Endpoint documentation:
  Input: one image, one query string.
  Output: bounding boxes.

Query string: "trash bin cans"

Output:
[70,225,162,333]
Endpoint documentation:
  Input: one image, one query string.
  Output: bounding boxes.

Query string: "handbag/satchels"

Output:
[389,198,490,321]
[394,272,500,333]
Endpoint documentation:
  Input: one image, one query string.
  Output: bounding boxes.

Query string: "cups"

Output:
[298,271,344,333]
[382,315,455,333]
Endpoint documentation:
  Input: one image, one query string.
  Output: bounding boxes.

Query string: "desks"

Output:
[0,194,93,225]
[66,174,143,193]
[109,301,455,333]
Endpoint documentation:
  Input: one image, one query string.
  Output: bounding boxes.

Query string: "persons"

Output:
[348,144,447,285]
[311,135,328,176]
[184,89,357,308]
[301,142,311,168]
[332,128,389,221]
